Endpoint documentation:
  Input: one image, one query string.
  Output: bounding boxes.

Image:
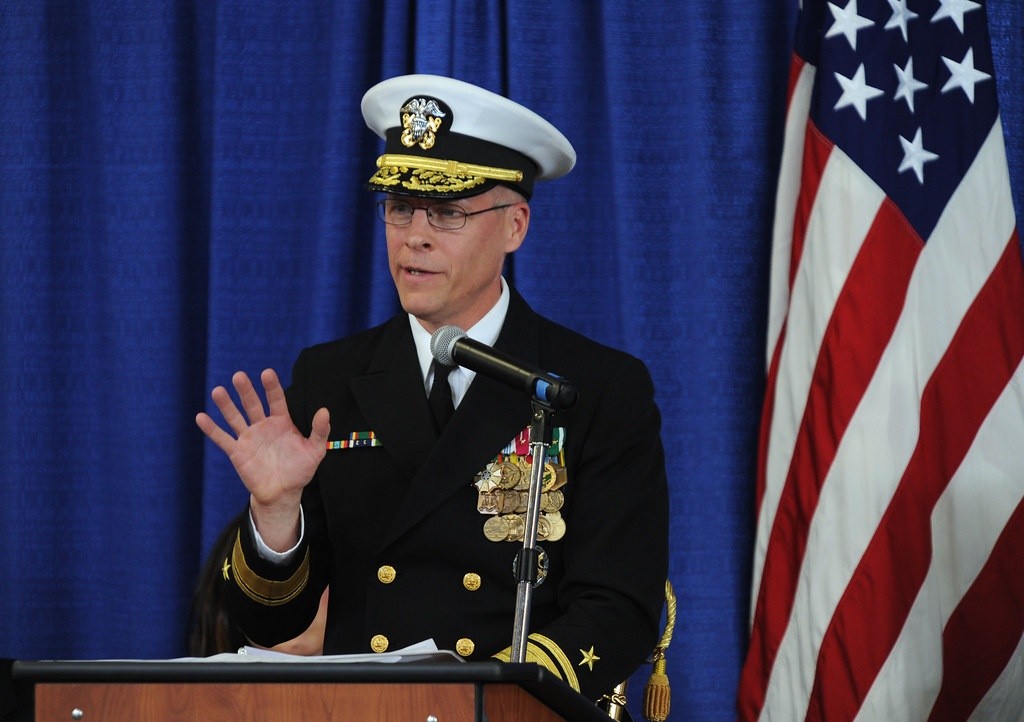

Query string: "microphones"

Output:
[430,325,578,410]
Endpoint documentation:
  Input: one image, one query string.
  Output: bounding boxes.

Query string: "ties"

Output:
[428,358,459,439]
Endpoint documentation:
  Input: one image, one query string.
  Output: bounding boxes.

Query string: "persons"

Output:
[196,73,671,703]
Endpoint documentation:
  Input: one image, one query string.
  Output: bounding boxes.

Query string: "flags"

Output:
[736,0,1024,722]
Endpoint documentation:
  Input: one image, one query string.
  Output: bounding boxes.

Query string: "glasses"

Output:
[377,198,516,230]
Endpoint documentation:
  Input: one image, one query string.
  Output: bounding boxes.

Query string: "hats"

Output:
[362,74,576,202]
